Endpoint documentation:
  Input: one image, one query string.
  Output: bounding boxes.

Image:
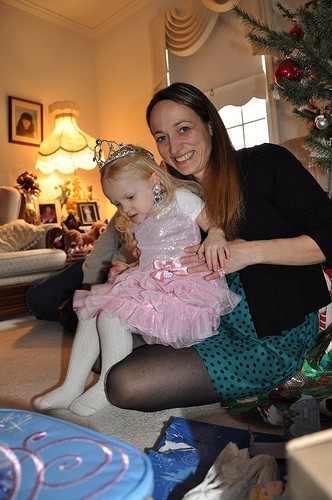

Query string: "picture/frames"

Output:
[39,204,58,224]
[77,202,100,225]
[8,95,44,147]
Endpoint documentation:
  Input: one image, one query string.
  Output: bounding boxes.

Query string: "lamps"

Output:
[35,100,103,177]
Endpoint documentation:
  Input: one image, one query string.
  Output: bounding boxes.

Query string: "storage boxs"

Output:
[0,409,154,500]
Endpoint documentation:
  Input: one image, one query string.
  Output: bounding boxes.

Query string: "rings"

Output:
[218,269,224,276]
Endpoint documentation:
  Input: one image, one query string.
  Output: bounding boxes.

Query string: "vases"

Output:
[22,201,38,221]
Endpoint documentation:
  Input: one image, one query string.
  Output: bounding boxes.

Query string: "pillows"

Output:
[0,219,38,253]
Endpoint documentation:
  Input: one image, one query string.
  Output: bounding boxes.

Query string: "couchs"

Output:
[0,186,67,320]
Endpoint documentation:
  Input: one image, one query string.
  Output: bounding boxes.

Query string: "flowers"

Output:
[56,178,94,215]
[16,172,41,197]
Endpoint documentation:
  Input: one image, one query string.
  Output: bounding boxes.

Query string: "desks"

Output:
[285,429,332,500]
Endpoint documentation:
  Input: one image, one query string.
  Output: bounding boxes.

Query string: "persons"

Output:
[104,83,332,411]
[84,206,93,222]
[41,206,56,223]
[38,143,231,417]
[16,113,36,138]
